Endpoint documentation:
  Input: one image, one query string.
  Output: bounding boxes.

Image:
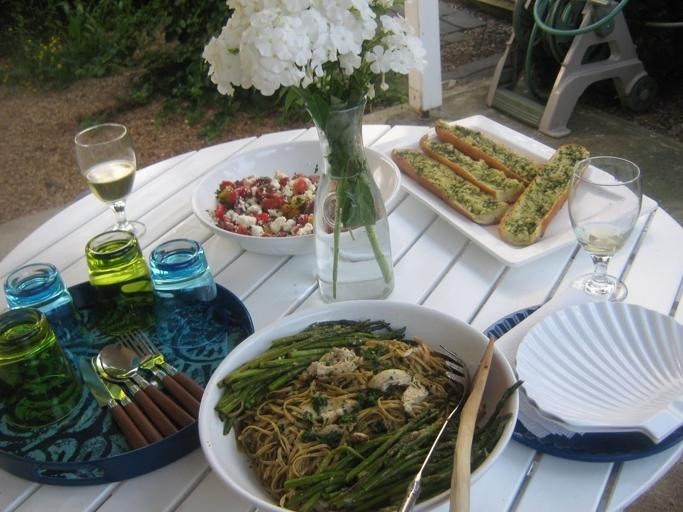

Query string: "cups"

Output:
[0,305,84,434]
[82,228,159,339]
[4,263,91,370]
[146,238,227,351]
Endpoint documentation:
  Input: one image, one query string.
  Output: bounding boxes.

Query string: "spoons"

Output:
[94,342,195,437]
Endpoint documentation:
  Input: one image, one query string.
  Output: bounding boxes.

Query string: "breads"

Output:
[390,118,590,247]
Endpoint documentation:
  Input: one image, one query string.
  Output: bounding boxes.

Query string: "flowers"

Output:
[201,0,427,301]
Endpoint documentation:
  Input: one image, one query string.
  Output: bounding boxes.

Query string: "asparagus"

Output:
[283,379,524,512]
[215,319,408,435]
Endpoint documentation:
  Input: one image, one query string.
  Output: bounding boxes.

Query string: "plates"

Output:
[480,305,683,464]
[368,112,617,268]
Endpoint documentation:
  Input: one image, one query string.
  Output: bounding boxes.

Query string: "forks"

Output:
[114,329,205,420]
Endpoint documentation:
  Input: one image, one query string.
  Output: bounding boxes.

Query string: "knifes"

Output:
[78,353,164,450]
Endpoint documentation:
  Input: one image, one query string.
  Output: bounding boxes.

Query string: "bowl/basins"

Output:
[189,139,401,257]
[194,297,523,512]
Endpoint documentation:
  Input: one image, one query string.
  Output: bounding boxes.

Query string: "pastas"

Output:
[238,336,463,504]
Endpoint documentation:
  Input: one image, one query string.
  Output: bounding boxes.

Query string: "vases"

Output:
[300,98,394,303]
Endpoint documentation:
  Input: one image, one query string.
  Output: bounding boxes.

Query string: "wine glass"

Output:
[71,121,151,241]
[570,154,644,304]
